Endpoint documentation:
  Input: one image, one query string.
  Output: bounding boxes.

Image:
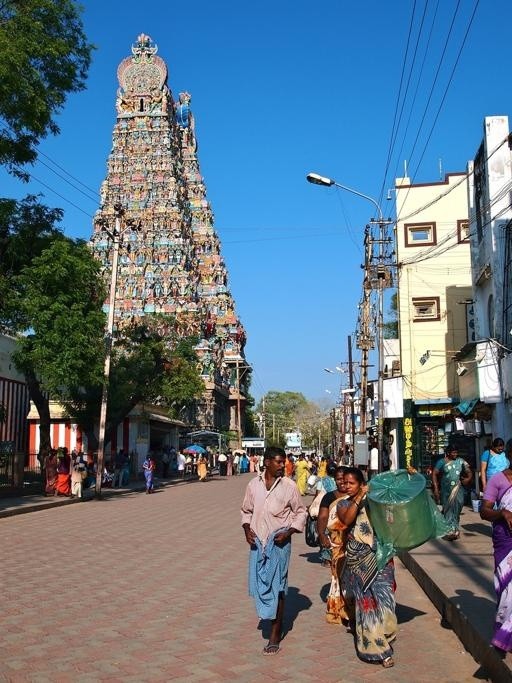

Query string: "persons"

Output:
[143,454,157,494]
[432,444,472,541]
[336,468,421,668]
[44,446,131,497]
[480,438,512,660]
[306,462,338,568]
[481,438,510,511]
[241,448,309,656]
[317,466,351,626]
[159,445,347,499]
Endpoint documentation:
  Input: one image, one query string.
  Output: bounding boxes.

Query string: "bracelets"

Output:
[354,500,360,507]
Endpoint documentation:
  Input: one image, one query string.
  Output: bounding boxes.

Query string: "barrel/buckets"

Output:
[366,470,434,550]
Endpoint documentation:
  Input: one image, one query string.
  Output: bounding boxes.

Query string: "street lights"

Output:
[305,170,384,473]
[323,363,357,396]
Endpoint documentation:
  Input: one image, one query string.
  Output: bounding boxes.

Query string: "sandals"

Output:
[447,534,453,541]
[451,531,460,538]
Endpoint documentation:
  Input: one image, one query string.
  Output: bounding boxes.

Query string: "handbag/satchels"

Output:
[479,475,487,492]
[309,477,325,519]
[436,499,443,512]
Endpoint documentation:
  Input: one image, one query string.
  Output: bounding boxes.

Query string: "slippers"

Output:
[263,645,280,656]
[383,656,394,668]
[345,625,352,633]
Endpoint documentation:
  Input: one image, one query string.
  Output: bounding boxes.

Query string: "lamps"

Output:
[423,350,461,359]
[456,360,476,376]
[419,355,457,365]
